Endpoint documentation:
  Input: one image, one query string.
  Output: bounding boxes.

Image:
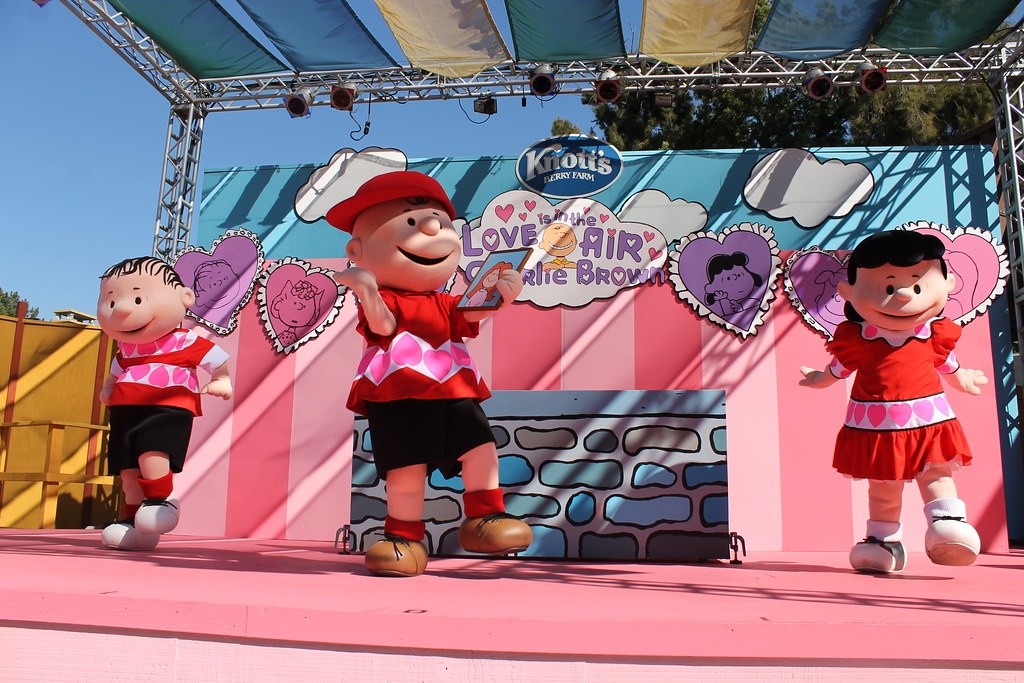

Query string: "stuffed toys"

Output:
[324,171,534,575]
[798,230,989,573]
[96,256,232,551]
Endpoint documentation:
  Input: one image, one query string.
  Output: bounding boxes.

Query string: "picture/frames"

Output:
[454,247,534,311]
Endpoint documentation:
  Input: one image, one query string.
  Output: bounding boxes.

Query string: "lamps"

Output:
[330,82,360,112]
[854,61,888,95]
[802,68,835,101]
[283,86,315,119]
[654,91,677,108]
[594,69,622,104]
[528,64,556,97]
